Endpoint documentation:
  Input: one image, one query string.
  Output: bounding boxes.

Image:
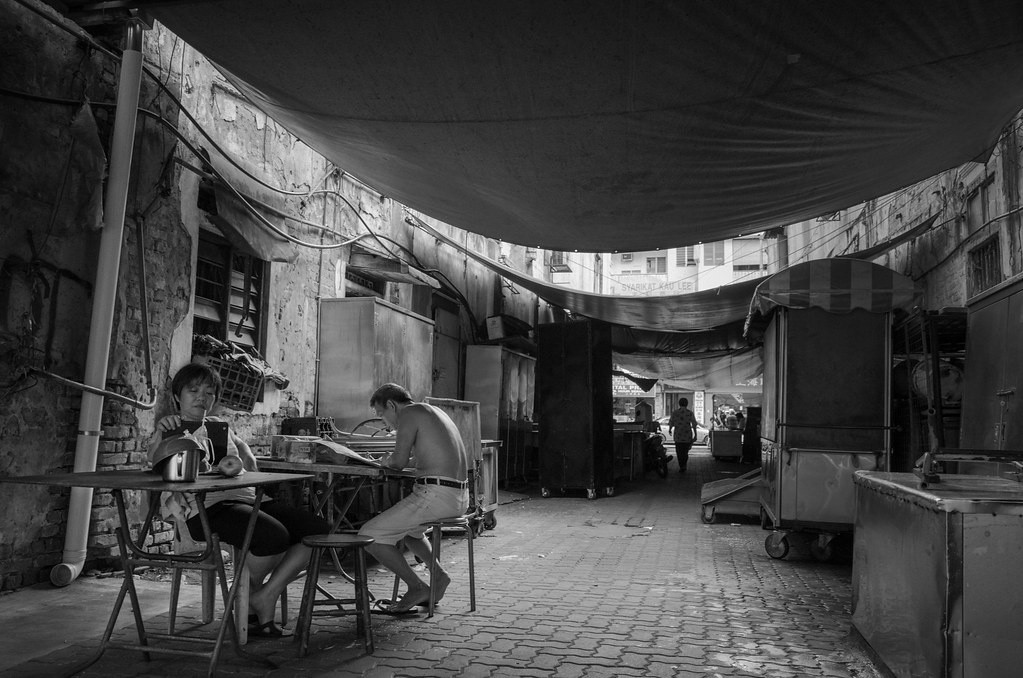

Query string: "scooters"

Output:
[630,409,672,479]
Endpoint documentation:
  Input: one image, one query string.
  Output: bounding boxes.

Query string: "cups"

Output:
[163,450,201,483]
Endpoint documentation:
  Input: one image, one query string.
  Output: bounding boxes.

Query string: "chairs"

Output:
[166,491,288,645]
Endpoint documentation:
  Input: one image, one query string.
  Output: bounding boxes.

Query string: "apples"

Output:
[218,455,243,477]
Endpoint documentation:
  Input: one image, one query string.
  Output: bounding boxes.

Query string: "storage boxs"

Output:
[486,316,529,340]
[193,354,264,413]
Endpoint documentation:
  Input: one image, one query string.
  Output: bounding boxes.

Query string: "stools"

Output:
[393,517,475,617]
[292,534,376,656]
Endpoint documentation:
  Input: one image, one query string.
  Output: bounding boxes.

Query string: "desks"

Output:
[0,468,316,678]
[257,459,415,628]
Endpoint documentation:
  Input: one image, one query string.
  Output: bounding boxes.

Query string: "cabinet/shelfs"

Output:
[537,318,616,500]
[319,296,436,512]
[465,344,537,489]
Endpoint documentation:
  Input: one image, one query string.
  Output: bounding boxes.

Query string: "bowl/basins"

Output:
[151,432,206,474]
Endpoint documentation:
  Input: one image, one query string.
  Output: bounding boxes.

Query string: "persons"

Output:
[667,398,698,472]
[147,360,326,638]
[730,412,746,434]
[358,383,469,613]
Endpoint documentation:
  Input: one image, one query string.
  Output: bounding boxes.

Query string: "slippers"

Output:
[398,594,438,606]
[248,620,293,638]
[248,613,259,624]
[370,598,418,615]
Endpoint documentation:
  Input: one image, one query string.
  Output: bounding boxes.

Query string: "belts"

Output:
[416,478,468,489]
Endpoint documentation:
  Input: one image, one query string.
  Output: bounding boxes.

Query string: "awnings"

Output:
[202,145,299,264]
[349,252,443,289]
